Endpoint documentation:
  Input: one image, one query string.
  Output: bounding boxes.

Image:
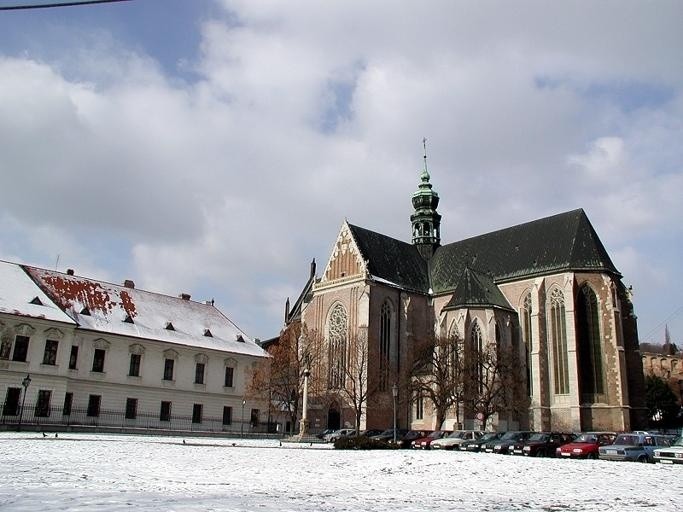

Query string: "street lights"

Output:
[391,381,399,444]
[17,374,31,432]
[651,408,662,432]
[302,369,310,419]
[239,395,246,433]
